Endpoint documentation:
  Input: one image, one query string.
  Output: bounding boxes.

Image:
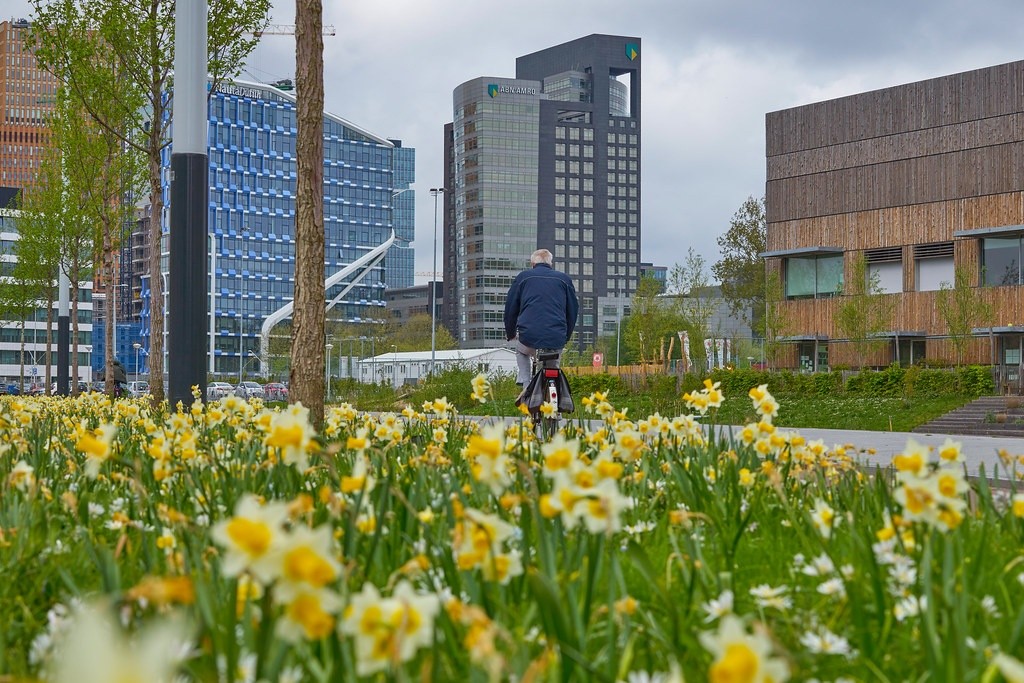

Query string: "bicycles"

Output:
[506,338,563,444]
[113,381,121,401]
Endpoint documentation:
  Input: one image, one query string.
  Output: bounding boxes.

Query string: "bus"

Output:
[127,381,149,391]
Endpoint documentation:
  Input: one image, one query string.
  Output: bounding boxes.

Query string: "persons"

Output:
[98,356,128,401]
[503,248,580,407]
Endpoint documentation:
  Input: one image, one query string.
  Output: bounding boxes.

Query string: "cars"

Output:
[0,381,106,397]
[207,382,236,400]
[236,381,265,401]
[265,383,288,399]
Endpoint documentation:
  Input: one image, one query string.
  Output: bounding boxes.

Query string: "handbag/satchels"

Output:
[526,367,575,414]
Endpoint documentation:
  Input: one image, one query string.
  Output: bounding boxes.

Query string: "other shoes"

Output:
[515,387,528,407]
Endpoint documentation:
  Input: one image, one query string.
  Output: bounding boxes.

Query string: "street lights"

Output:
[133,342,141,398]
[429,187,449,385]
[248,350,268,381]
[85,345,92,390]
[391,344,398,366]
[615,272,624,377]
[325,343,334,399]
